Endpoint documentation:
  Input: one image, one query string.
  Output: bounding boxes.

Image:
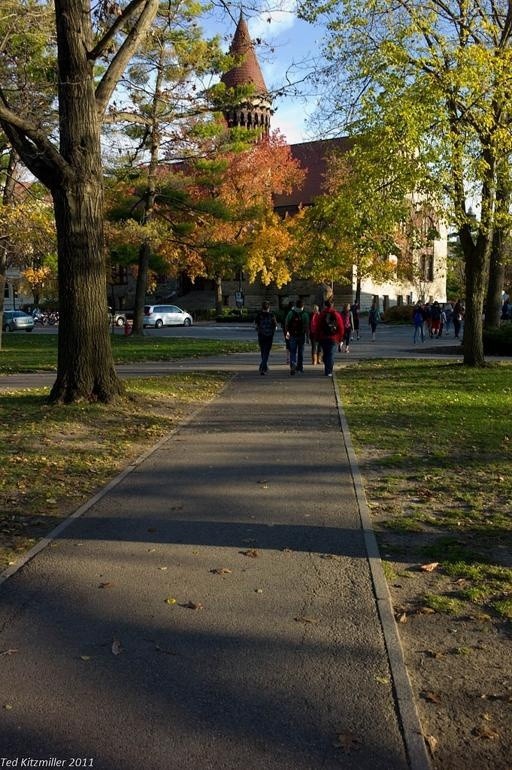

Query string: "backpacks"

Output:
[343,313,351,329]
[288,310,305,336]
[320,313,338,336]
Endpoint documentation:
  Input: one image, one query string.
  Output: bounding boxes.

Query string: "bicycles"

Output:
[33,312,59,326]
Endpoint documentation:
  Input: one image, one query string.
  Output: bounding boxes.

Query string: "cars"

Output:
[107,306,126,326]
[2,310,34,332]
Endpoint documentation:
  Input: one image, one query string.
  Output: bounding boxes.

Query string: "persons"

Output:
[500,303,508,320]
[254,300,277,377]
[314,298,345,377]
[284,299,310,375]
[275,300,360,364]
[412,299,464,345]
[368,303,380,341]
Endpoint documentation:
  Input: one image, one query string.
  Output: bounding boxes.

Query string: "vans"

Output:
[143,305,194,328]
